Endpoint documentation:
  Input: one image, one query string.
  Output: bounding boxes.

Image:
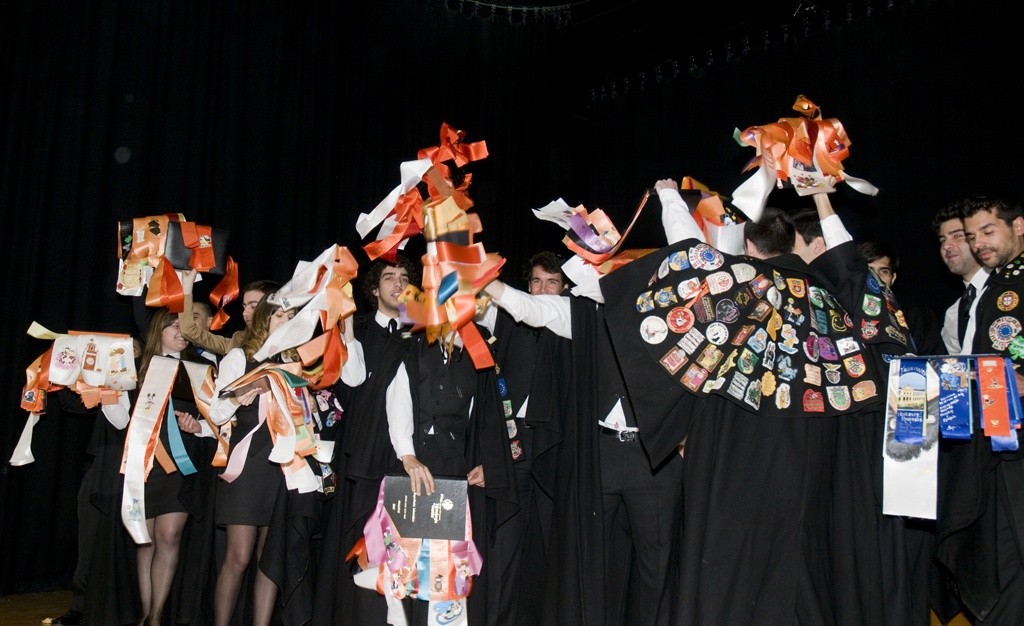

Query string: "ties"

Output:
[958,283,976,349]
[388,317,398,333]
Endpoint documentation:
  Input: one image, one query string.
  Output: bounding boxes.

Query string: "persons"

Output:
[41,179,1024,626]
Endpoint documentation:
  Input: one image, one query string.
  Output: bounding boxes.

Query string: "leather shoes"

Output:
[51,608,83,626]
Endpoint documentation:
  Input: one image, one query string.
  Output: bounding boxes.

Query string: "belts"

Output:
[600,428,642,443]
